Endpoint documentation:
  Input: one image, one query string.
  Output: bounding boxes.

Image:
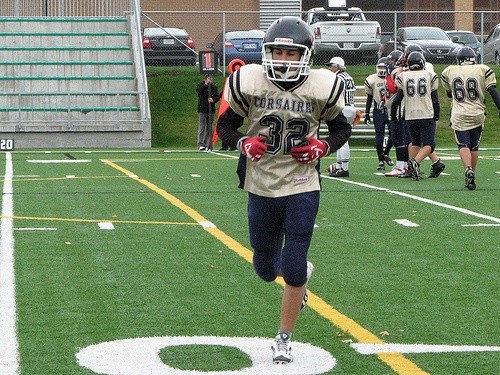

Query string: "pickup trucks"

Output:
[302,6,381,66]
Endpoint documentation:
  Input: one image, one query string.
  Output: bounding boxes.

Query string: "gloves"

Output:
[242,136,266,162]
[365,114,371,126]
[291,137,328,165]
[391,117,398,125]
[435,116,440,121]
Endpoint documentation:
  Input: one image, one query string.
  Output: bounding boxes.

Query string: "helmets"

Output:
[262,17,315,81]
[377,57,387,76]
[398,53,408,67]
[387,50,403,74]
[404,44,423,59]
[408,52,426,70]
[457,46,476,65]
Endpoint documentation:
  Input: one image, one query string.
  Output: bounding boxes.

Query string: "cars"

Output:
[206,29,267,67]
[141,27,197,66]
[441,30,483,54]
[383,26,462,65]
[478,24,500,66]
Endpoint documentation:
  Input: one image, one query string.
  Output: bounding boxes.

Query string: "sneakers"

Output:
[465,167,477,190]
[300,261,314,311]
[407,160,419,181]
[329,168,349,178]
[271,332,293,365]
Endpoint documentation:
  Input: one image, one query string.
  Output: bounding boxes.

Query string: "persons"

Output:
[383,45,445,182]
[341,106,362,125]
[441,46,500,190]
[363,57,394,171]
[196,74,219,153]
[325,56,354,177]
[218,91,243,151]
[217,15,351,365]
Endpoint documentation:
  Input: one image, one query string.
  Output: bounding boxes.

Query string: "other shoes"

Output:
[219,147,234,151]
[384,154,394,166]
[377,160,386,171]
[428,158,445,178]
[398,170,410,178]
[385,166,408,177]
[199,146,208,151]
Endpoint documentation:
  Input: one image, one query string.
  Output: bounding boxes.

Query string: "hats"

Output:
[324,57,345,67]
